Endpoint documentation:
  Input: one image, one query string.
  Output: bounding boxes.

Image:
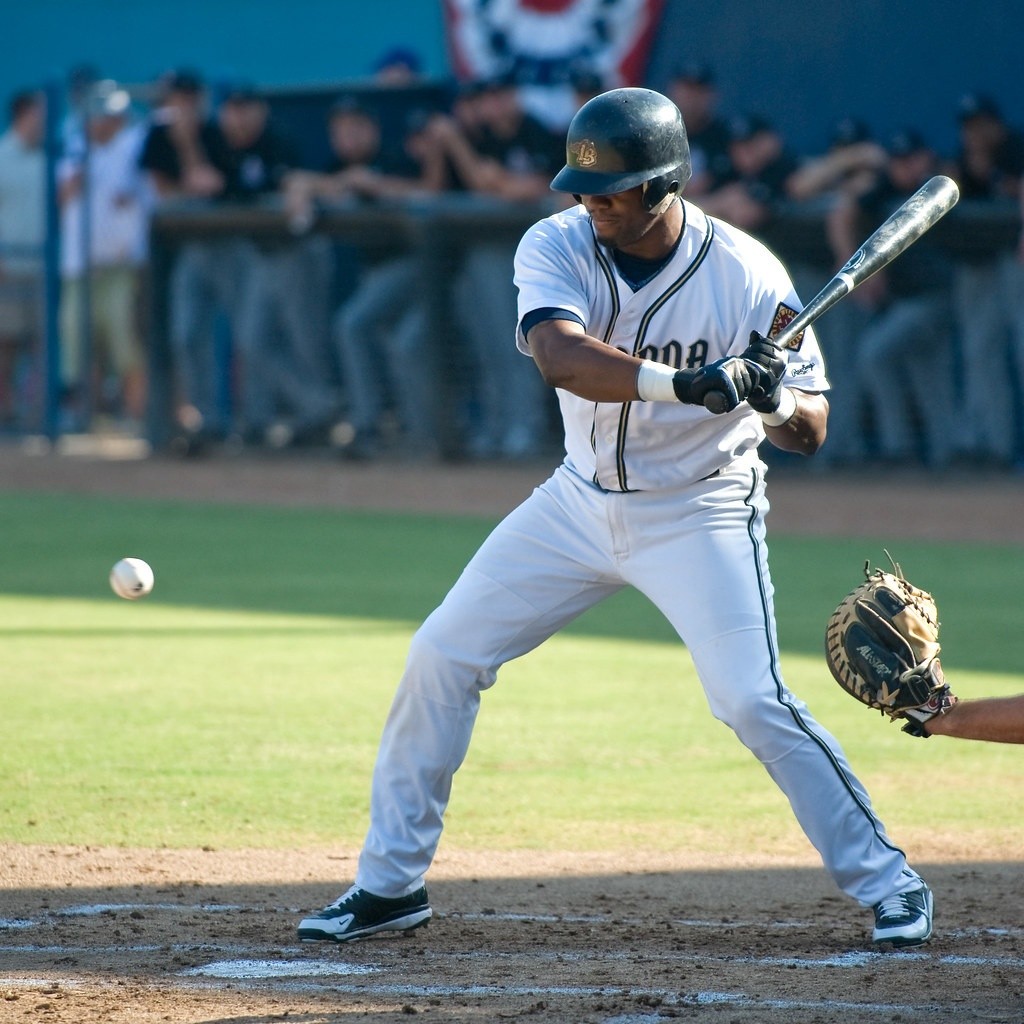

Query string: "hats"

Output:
[371,50,417,71]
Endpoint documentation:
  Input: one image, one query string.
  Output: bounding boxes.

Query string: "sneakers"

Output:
[871,883,934,949]
[295,884,432,944]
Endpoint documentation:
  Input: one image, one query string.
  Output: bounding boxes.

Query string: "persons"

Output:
[298,88,933,945]
[671,66,1024,460]
[0,86,152,431]
[825,569,1024,744]
[141,51,610,467]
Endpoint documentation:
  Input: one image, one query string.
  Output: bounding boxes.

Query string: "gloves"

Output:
[672,355,765,414]
[740,330,789,414]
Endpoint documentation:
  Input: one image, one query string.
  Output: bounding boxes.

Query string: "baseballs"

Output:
[110,557,155,601]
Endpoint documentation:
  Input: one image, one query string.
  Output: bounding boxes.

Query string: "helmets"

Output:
[549,86,693,217]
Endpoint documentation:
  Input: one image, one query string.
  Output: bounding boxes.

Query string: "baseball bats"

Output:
[704,173,961,415]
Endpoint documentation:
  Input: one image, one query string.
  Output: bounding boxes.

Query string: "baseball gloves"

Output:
[823,567,957,742]
